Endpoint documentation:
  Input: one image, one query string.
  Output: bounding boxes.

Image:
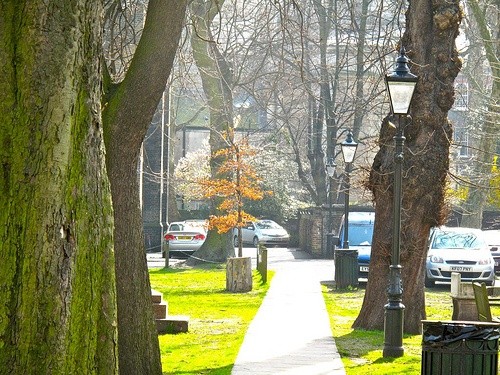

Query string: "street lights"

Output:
[338,129,357,249]
[325,157,337,258]
[382,45,418,361]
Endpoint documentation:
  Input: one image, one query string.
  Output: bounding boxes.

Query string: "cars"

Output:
[480,229,500,273]
[233,219,290,249]
[185,218,209,234]
[162,222,208,259]
[425,227,496,288]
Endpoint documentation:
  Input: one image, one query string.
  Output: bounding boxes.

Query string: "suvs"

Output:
[336,211,378,277]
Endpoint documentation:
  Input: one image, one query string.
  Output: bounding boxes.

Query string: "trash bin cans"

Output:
[420,320,500,375]
[334,249,360,291]
